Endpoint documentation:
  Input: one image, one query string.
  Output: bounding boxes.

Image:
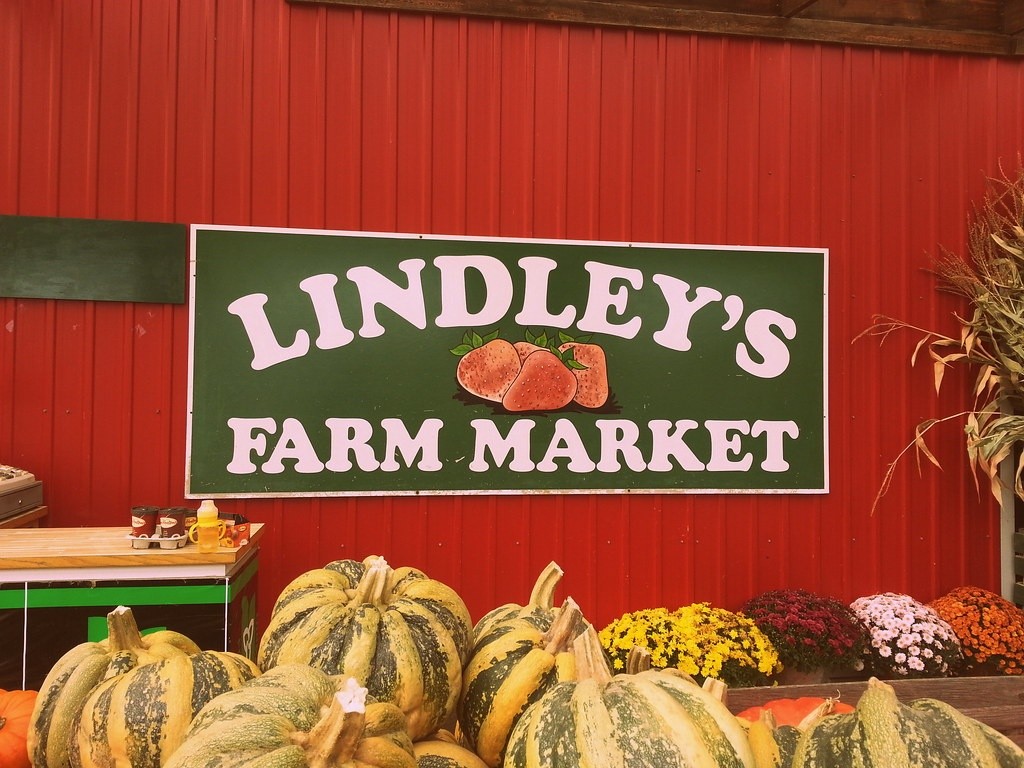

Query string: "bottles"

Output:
[189,499,226,552]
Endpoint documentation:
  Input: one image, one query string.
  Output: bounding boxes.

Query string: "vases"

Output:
[778,664,825,686]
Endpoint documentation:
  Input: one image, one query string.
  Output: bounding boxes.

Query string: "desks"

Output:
[0,522,266,692]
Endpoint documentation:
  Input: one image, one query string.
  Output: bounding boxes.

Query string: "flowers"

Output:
[598,602,784,687]
[739,588,871,671]
[850,592,965,677]
[927,586,1024,675]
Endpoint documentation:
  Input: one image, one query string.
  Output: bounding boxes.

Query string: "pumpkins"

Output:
[0,554,1024,768]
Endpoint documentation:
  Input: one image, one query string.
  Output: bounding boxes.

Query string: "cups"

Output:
[158,508,184,537]
[131,506,158,538]
